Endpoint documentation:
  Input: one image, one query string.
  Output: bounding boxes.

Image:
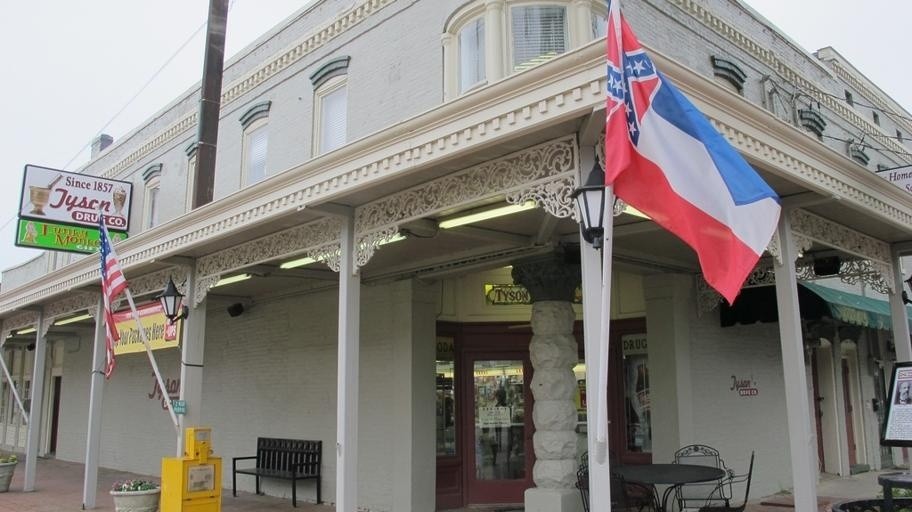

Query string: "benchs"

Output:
[229,436,323,507]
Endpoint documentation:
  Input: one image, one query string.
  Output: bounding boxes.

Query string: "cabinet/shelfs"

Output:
[436,368,457,459]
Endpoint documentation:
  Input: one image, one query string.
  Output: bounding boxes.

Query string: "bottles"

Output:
[635,362,652,441]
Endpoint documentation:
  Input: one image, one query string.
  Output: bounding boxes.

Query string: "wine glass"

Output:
[112,192,127,218]
[29,185,51,215]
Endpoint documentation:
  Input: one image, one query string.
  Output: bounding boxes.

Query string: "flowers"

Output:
[115,478,161,495]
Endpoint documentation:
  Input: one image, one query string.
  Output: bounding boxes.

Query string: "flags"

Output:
[98,210,131,378]
[605,0,784,308]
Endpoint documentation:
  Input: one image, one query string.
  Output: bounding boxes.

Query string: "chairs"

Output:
[574,441,756,512]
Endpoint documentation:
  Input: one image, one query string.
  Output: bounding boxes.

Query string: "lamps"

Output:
[154,274,191,329]
[567,153,607,253]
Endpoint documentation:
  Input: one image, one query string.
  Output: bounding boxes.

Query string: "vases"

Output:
[111,487,161,511]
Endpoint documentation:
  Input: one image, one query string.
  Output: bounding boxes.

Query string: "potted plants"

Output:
[0,453,19,494]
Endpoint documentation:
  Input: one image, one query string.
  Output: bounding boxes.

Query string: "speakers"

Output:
[27,343,35,350]
[813,249,840,275]
[228,303,243,317]
